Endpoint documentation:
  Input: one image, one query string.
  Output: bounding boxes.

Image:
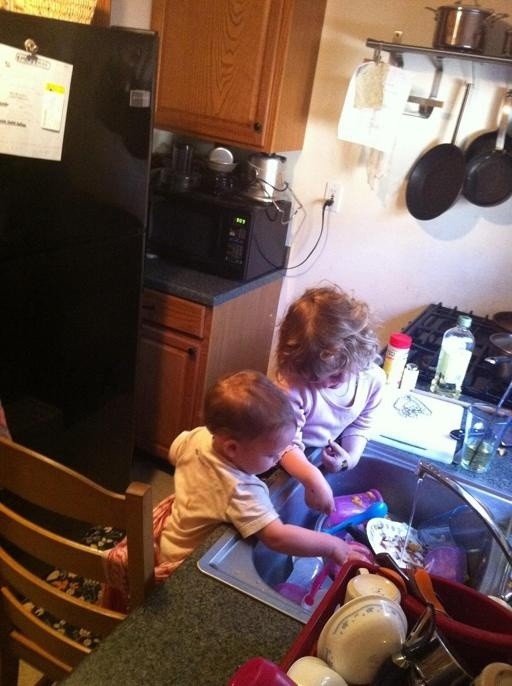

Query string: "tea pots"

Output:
[191,150,259,196]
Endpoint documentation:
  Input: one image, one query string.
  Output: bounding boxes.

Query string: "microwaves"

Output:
[148,186,292,285]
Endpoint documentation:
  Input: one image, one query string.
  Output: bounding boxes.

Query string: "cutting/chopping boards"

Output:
[368,386,463,465]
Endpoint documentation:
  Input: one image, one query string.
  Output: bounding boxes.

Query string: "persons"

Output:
[264,278,388,513]
[98,367,378,618]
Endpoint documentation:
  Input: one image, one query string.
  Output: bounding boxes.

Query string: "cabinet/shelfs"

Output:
[129,262,285,465]
[150,0,327,156]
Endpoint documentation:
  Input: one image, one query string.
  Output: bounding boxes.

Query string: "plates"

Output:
[365,518,425,571]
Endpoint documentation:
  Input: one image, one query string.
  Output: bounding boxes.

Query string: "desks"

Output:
[0,186,143,441]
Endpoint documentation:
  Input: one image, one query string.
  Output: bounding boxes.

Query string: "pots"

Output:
[426,0,502,49]
[484,328,512,388]
[406,85,472,222]
[462,91,510,207]
[493,311,512,334]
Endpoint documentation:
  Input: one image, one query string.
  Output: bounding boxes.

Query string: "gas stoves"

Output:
[378,302,512,405]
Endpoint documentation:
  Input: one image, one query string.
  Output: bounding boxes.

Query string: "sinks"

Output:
[197,439,512,646]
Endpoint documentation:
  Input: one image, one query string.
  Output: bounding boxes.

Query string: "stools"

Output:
[1,404,153,685]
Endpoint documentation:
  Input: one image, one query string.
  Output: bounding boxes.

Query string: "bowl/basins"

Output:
[313,597,409,685]
[415,627,475,686]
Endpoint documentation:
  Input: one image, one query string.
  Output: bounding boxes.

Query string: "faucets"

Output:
[415,460,512,562]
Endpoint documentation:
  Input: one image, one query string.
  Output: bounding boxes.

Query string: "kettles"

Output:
[242,151,289,205]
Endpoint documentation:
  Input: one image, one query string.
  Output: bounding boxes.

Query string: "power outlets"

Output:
[323,182,342,212]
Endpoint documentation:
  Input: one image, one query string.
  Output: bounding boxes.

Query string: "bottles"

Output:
[428,314,475,401]
[382,333,413,387]
[461,404,509,476]
[400,363,418,388]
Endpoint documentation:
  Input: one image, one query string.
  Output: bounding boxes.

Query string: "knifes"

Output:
[449,422,512,447]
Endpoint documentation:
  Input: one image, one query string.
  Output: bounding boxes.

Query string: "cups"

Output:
[282,657,348,686]
[419,505,487,552]
[342,565,401,609]
[169,142,194,176]
